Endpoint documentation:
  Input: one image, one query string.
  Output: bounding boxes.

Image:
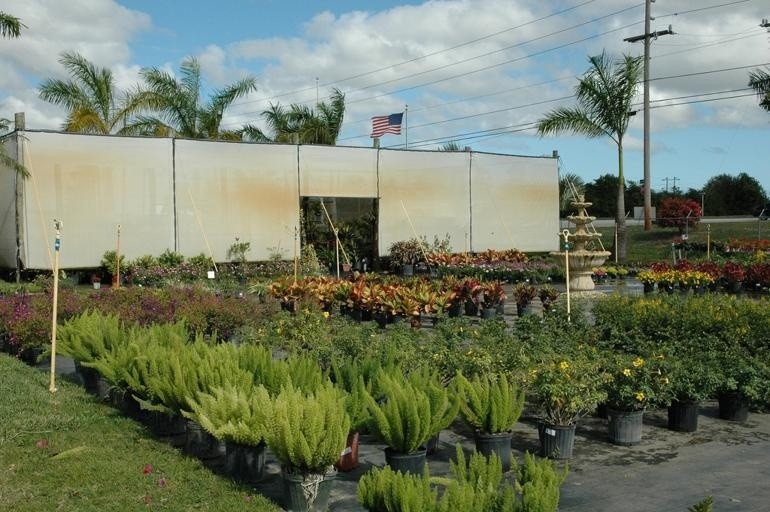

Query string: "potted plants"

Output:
[0,232,770,512]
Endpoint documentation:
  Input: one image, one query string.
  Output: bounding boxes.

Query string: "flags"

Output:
[369,112,404,138]
